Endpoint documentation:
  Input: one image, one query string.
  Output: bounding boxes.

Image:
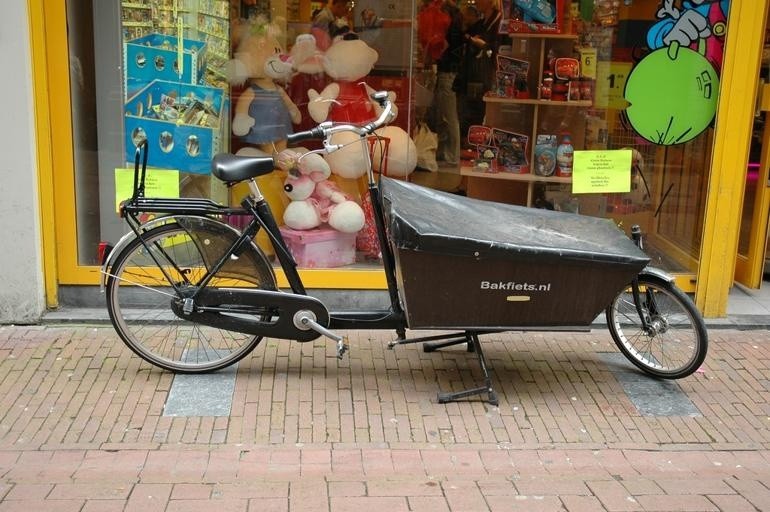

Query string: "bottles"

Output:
[557,135,573,177]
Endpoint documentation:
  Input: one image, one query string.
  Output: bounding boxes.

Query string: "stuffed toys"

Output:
[224,13,417,261]
[223,13,418,260]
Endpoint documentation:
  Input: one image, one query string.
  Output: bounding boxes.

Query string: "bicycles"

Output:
[97,90,708,408]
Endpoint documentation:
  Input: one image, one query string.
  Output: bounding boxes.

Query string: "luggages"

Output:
[355,137,391,261]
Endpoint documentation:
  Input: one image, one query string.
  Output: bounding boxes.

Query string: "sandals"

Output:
[448,185,467,197]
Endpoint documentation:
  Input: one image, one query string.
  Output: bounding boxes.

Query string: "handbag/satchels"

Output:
[467,83,483,101]
[414,132,438,172]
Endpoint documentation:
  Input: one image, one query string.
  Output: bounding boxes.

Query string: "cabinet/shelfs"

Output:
[455,33,596,214]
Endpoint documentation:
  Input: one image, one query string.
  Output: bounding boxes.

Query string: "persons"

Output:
[311,1,348,34]
[419,2,496,169]
